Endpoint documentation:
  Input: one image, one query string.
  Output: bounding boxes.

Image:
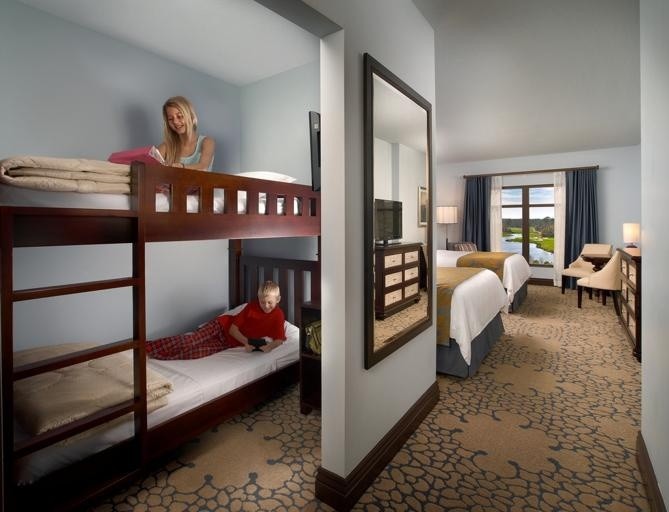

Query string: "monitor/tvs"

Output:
[309,110,321,192]
[375,199,402,245]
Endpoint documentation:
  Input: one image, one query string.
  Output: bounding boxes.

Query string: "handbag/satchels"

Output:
[304,321,320,354]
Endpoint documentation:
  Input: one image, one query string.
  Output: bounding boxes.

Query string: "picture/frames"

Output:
[417,185,427,229]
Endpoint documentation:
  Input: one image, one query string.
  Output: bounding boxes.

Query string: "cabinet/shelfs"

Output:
[376,241,423,321]
[617,248,642,363]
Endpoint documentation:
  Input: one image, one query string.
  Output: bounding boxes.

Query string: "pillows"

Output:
[229,170,298,198]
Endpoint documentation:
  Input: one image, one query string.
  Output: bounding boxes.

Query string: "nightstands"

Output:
[299,300,324,414]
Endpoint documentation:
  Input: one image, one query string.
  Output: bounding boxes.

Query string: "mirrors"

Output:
[361,53,434,369]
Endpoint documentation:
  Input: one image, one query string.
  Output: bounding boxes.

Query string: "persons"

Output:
[144,279,286,361]
[156,96,217,194]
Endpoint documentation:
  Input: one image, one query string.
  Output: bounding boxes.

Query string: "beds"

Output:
[437,249,532,312]
[0,156,318,512]
[437,266,507,379]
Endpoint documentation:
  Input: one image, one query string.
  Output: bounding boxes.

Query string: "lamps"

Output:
[621,222,640,248]
[437,206,458,250]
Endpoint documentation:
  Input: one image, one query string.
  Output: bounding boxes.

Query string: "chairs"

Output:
[447,241,478,253]
[563,244,622,317]
